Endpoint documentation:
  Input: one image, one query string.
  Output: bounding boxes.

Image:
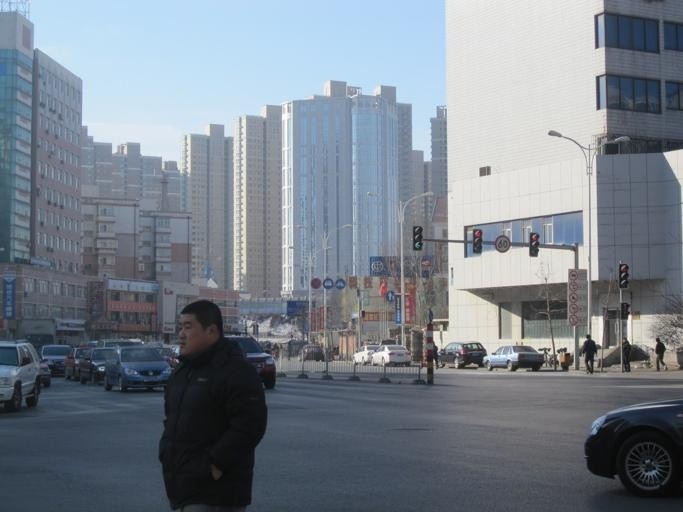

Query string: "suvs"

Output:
[436,341,488,369]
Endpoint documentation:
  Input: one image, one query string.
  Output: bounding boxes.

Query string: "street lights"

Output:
[286,245,333,345]
[295,223,353,348]
[546,128,631,342]
[366,190,434,347]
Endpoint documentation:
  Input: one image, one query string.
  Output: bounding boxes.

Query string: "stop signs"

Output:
[566,268,589,328]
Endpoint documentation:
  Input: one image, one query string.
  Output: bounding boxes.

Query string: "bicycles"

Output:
[538,346,559,369]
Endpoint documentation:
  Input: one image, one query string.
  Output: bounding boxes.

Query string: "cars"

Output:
[579,396,682,499]
[480,344,545,373]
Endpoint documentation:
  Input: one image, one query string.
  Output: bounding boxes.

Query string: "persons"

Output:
[580,334,598,375]
[433,341,439,369]
[654,338,668,371]
[155,299,270,512]
[622,337,632,372]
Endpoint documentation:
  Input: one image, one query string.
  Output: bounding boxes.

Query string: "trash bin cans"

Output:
[560,353,570,371]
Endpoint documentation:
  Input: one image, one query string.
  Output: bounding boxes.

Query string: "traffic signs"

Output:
[494,234,510,253]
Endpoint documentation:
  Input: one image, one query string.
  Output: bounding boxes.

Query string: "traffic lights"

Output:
[411,225,423,250]
[618,262,629,289]
[471,228,483,253]
[618,302,631,321]
[527,231,539,258]
